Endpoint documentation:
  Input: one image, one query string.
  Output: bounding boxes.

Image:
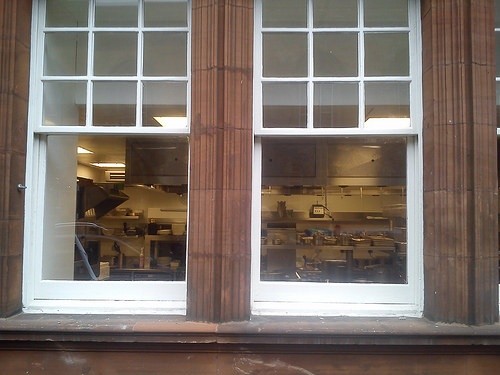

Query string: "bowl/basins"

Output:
[262,236,267,244]
[273,239,281,244]
[300,236,312,244]
[103,228,114,235]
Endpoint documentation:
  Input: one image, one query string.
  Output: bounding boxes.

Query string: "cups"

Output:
[119,253,125,268]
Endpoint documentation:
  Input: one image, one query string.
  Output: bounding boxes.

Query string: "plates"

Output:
[156,229,171,235]
[157,256,170,266]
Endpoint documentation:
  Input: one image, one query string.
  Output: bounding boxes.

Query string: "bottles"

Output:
[138,248,144,268]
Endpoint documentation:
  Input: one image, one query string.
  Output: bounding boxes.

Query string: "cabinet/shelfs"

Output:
[85,208,141,240]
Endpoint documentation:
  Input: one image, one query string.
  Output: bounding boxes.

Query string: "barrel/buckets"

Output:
[313,233,325,246]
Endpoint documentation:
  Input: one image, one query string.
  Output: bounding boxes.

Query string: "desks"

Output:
[97,255,185,281]
[299,241,395,272]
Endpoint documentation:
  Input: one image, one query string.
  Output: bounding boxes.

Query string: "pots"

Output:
[337,234,353,245]
